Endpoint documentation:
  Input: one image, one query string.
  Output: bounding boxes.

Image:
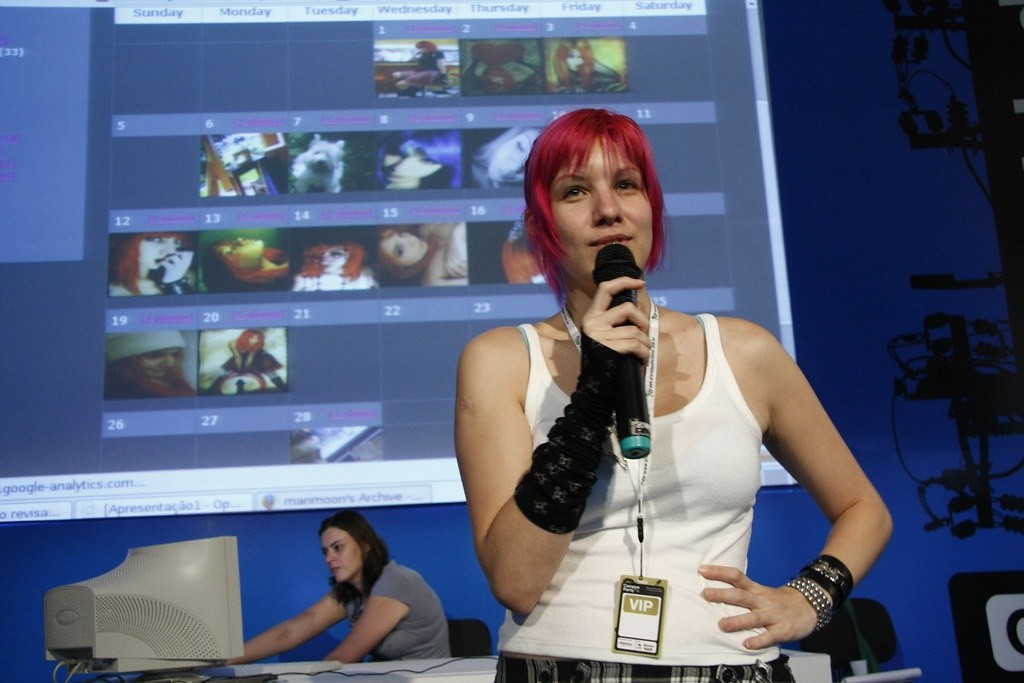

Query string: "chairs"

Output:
[447,618,499,683]
[799,598,905,683]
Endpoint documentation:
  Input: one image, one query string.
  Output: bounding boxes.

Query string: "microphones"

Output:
[592,243,655,453]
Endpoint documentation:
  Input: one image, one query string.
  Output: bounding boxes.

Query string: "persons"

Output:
[392,41,452,97]
[199,327,287,395]
[545,37,627,93]
[103,331,198,399]
[203,237,290,292]
[292,242,378,291]
[455,110,892,683]
[378,129,461,191]
[108,232,197,297]
[362,223,468,286]
[467,127,543,188]
[216,509,451,667]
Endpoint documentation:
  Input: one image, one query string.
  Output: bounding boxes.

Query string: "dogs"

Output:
[291,133,346,196]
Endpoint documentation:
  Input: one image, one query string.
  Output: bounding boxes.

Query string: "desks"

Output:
[109,655,499,683]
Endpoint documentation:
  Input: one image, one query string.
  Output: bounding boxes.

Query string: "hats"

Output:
[104,327,186,364]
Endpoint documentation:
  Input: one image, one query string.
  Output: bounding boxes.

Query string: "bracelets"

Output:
[785,576,834,633]
[799,553,853,612]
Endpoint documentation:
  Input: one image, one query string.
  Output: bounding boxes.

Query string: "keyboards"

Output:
[207,658,344,673]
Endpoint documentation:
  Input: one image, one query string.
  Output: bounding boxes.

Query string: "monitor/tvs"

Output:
[43,535,244,682]
